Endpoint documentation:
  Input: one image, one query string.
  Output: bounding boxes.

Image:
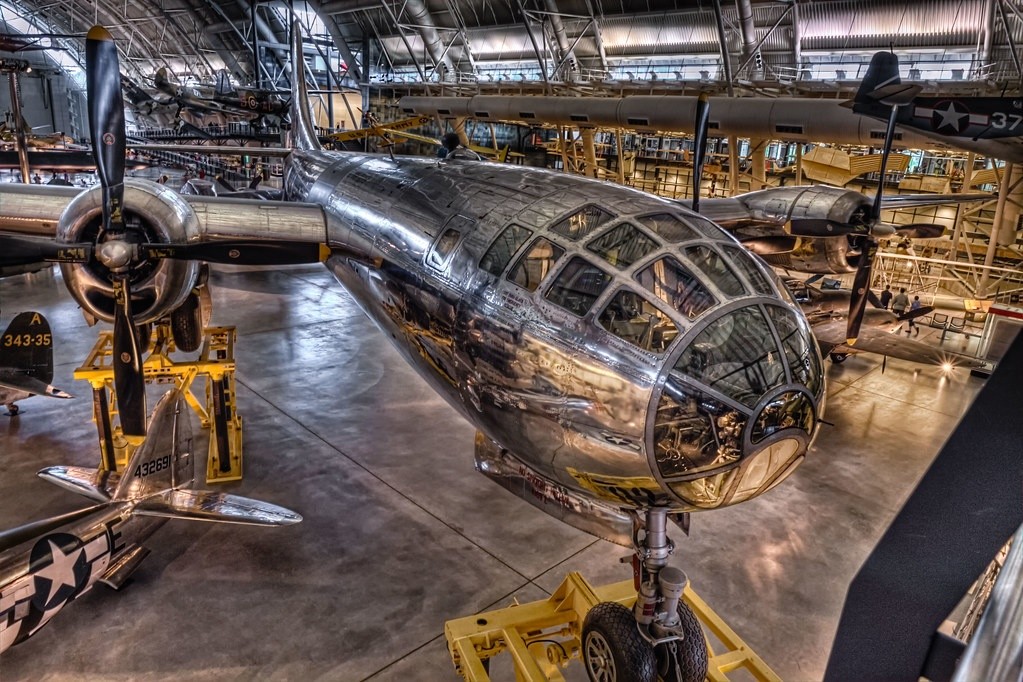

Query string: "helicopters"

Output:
[0,22,1023,682]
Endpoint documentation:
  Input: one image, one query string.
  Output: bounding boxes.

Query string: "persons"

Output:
[891,288,909,329]
[881,285,892,309]
[905,296,920,334]
